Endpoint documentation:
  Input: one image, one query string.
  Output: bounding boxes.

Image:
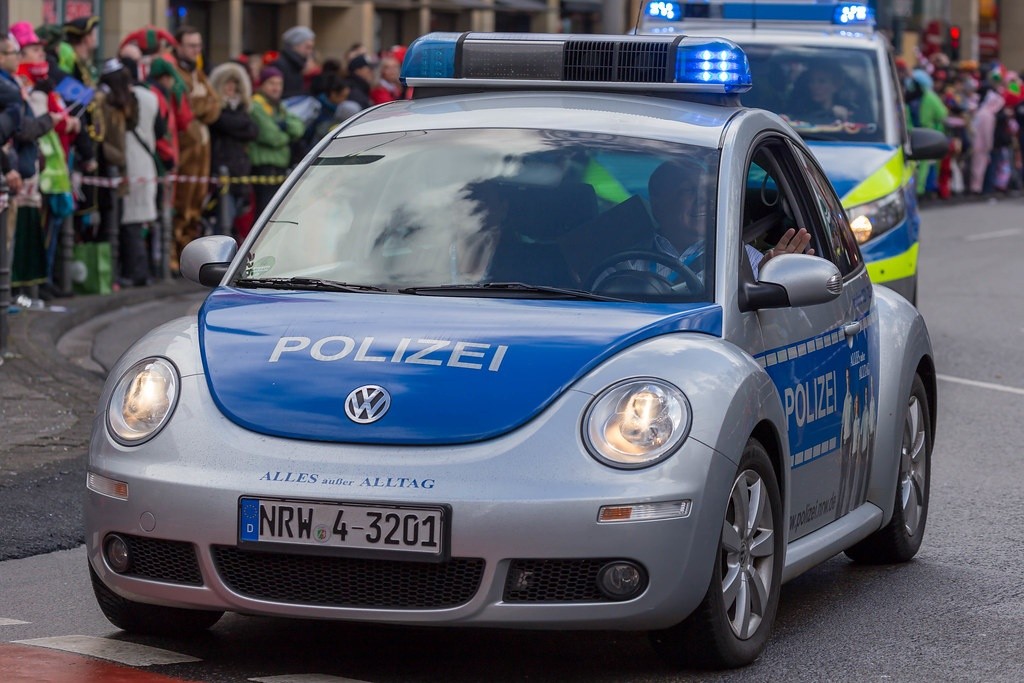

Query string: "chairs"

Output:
[488,180,597,296]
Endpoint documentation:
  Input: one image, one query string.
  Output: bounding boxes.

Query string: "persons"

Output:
[397,155,815,296]
[893,51,1024,209]
[745,53,863,123]
[0,13,414,313]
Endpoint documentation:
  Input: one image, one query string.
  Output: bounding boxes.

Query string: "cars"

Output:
[582,0,951,311]
[78,32,940,679]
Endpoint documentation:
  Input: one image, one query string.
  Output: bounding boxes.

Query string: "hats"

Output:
[348,52,379,74]
[62,14,100,46]
[35,24,66,49]
[6,22,50,50]
[281,25,316,46]
[257,64,284,86]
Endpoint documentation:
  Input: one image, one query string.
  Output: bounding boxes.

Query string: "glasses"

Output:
[0,48,24,57]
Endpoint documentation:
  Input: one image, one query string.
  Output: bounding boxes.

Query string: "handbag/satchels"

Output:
[73,242,113,297]
[153,151,167,177]
